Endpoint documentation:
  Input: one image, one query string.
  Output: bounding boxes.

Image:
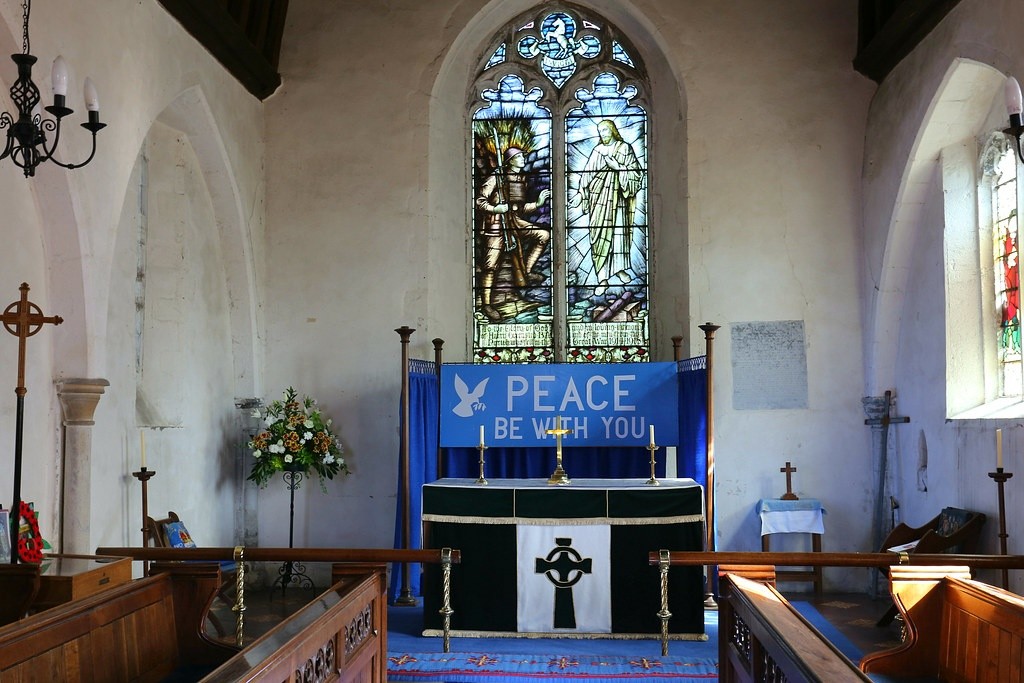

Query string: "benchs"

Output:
[0,563,244,683]
[859,565,1024,683]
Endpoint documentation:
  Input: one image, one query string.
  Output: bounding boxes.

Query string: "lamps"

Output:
[0,0,107,179]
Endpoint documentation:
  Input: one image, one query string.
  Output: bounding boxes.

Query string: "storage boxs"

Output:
[18,553,133,603]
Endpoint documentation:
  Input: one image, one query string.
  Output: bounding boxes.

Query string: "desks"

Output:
[755,498,828,601]
[419,477,710,641]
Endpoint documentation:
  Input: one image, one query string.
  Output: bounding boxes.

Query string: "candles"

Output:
[140,429,145,467]
[479,425,484,446]
[996,429,1002,468]
[650,425,654,447]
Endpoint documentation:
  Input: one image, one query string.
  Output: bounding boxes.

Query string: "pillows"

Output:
[162,521,196,549]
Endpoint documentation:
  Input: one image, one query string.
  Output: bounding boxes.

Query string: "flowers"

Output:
[232,385,352,498]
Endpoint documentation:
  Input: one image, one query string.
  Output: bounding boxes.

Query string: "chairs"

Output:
[875,505,987,628]
[148,511,249,639]
